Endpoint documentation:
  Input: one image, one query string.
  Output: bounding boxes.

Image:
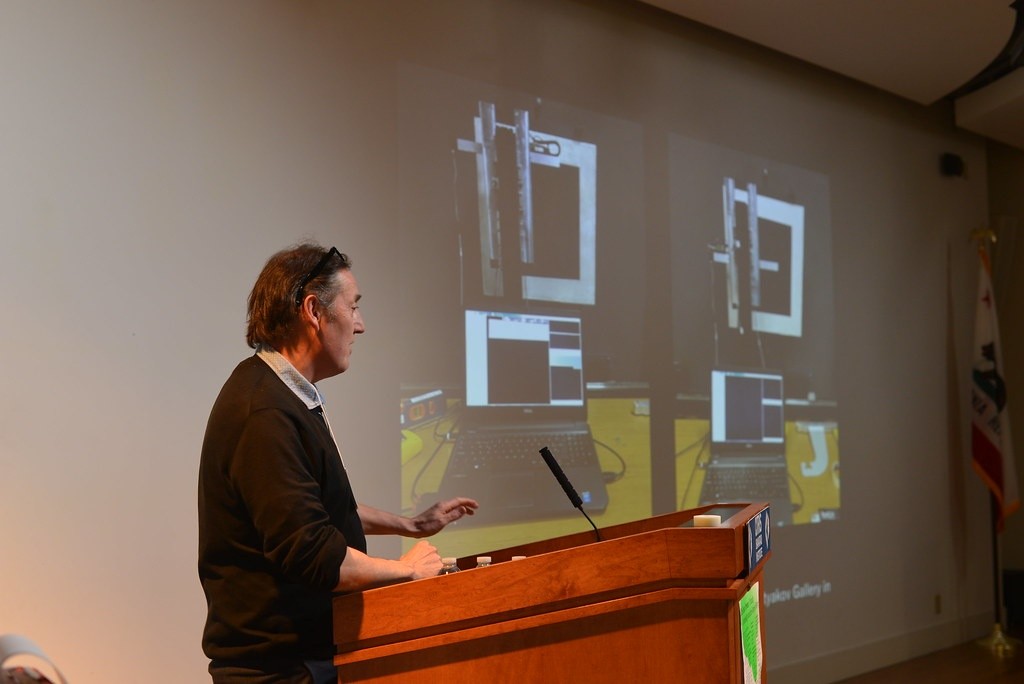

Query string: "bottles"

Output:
[439,556,530,577]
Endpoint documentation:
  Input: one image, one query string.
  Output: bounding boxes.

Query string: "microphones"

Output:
[539,446,601,542]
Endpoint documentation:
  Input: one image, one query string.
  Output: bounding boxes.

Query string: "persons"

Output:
[195,245,479,684]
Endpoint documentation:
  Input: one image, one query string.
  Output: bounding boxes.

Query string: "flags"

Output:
[970,250,1022,533]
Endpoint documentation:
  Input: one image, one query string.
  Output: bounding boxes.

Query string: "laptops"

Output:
[698,369,793,527]
[437,309,609,522]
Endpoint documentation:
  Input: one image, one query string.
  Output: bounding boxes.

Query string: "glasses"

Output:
[295,246,352,307]
